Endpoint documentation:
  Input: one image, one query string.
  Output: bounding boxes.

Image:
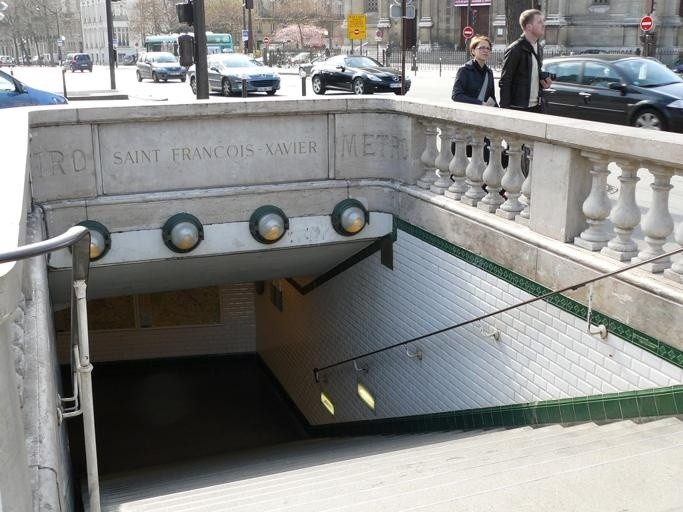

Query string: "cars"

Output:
[187,53,280,95]
[135,51,186,82]
[63,51,92,72]
[0,67,67,108]
[537,52,683,132]
[310,53,411,96]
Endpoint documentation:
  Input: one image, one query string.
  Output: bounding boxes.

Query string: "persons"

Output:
[481,6,555,200]
[450,34,499,192]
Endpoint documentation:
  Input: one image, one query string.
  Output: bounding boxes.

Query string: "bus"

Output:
[144,33,232,53]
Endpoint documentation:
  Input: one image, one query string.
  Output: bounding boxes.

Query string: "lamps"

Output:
[248,204,291,246]
[331,199,369,238]
[161,213,204,254]
[68,220,111,262]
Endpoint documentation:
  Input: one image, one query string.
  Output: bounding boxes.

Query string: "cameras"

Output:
[540,72,556,80]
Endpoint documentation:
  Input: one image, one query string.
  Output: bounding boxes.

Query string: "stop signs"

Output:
[463,27,474,38]
[640,16,653,32]
[263,36,269,44]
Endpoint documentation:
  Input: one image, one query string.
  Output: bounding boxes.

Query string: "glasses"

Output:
[475,45,491,51]
